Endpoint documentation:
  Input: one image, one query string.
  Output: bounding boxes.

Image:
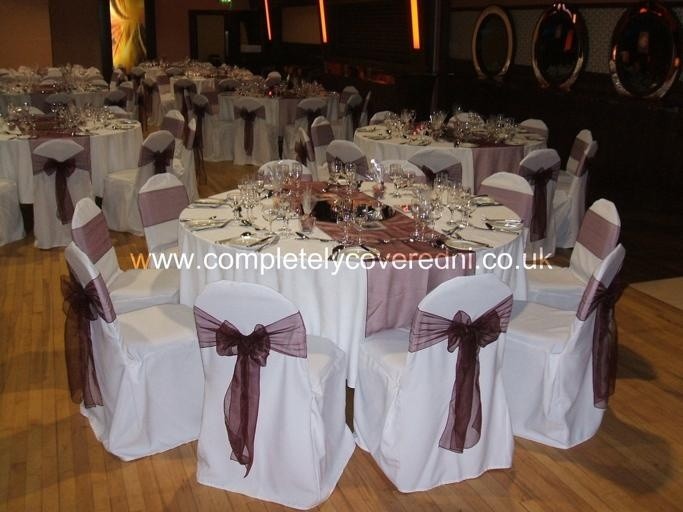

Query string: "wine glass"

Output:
[357,108,544,149]
[2,63,108,95]
[141,56,253,80]
[0,94,138,138]
[180,159,526,262]
[231,71,332,98]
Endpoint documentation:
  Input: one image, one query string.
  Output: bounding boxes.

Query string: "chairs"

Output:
[1,61,627,512]
